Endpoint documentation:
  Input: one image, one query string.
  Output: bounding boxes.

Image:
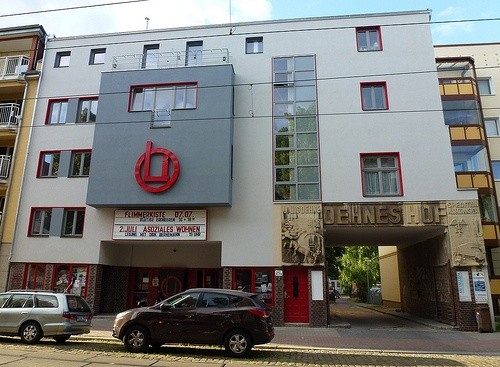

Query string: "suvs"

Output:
[112,288,275,358]
[329,287,335,303]
[0,288,94,343]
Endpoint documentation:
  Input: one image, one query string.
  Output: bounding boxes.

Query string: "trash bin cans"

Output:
[473,306,493,333]
